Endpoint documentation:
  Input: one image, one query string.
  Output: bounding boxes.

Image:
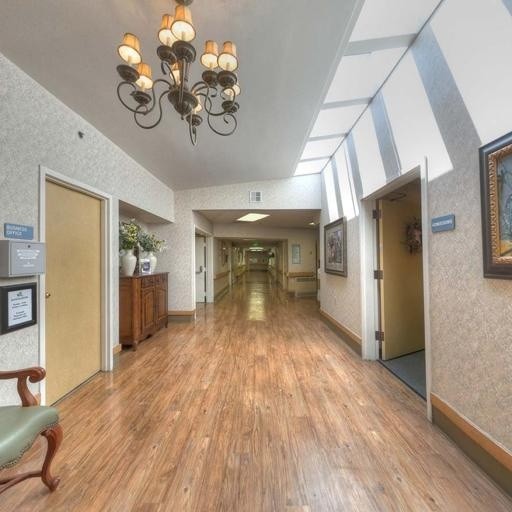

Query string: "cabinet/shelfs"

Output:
[120,271,171,351]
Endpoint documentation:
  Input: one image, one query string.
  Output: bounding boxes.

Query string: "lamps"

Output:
[114,1,245,146]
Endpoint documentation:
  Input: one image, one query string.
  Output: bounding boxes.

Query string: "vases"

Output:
[119,249,158,277]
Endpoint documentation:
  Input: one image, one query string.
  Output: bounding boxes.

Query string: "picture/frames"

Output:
[0,281,39,335]
[323,215,348,278]
[478,130,512,280]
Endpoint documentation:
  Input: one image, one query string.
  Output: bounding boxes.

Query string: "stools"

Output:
[0,362,64,498]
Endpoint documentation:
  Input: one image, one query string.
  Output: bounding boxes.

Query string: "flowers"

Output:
[401,216,424,255]
[120,214,167,253]
